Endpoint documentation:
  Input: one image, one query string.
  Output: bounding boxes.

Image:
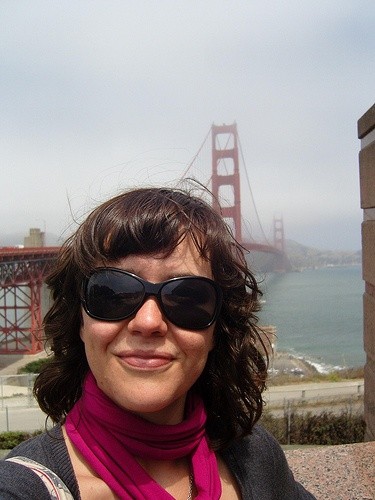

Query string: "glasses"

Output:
[80,267,221,331]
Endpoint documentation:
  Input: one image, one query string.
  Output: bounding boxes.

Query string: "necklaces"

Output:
[186,472,194,500]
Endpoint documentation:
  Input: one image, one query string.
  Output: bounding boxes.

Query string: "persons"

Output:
[0,177,319,500]
